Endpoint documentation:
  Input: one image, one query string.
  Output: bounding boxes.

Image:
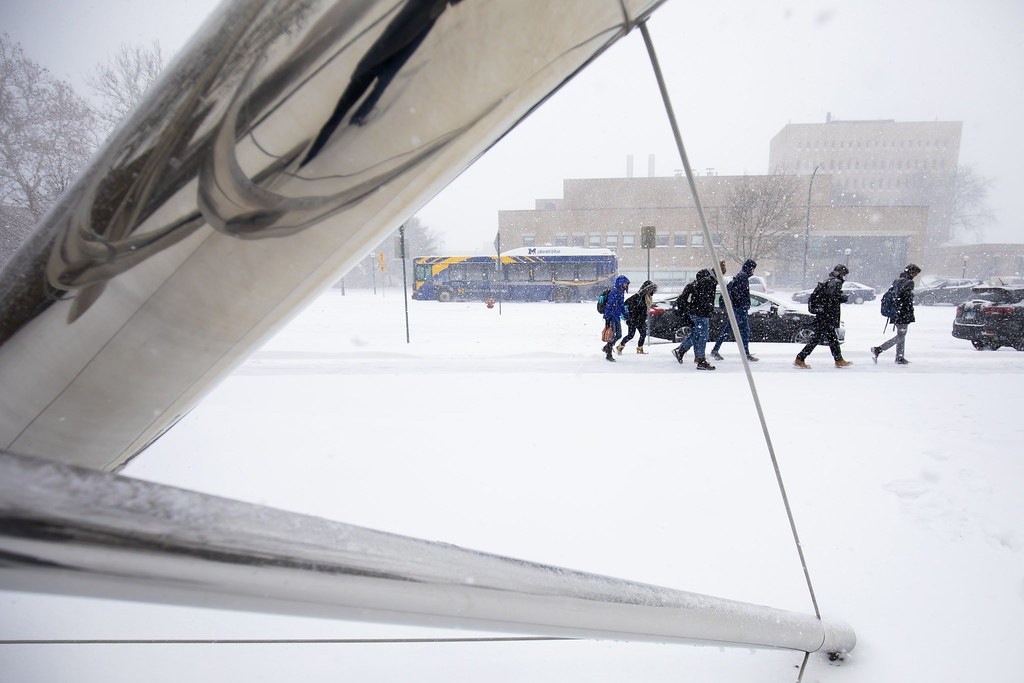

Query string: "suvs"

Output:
[911,278,1024,350]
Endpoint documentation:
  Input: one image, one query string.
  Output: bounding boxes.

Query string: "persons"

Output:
[602,275,630,361]
[711,258,758,362]
[793,264,853,368]
[871,264,922,364]
[673,261,726,370]
[613,280,657,355]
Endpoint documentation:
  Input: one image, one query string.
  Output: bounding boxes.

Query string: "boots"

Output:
[636,347,649,355]
[616,343,624,355]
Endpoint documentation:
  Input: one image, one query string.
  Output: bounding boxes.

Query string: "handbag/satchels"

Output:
[601,319,614,342]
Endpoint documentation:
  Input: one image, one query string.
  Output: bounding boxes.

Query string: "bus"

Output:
[412,246,620,303]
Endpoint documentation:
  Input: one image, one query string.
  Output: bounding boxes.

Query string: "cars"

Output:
[644,275,846,345]
[792,282,878,305]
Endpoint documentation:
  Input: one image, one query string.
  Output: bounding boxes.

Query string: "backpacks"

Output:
[671,281,697,315]
[881,278,910,317]
[808,277,839,314]
[596,287,618,314]
[719,273,745,315]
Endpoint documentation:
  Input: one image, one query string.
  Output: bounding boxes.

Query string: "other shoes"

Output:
[748,356,760,363]
[607,354,615,363]
[835,360,853,368]
[794,358,810,369]
[895,357,910,365]
[672,348,684,364]
[696,360,715,371]
[872,346,880,364]
[710,350,724,360]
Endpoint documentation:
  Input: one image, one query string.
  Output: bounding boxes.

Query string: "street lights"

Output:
[962,256,971,277]
[844,248,852,266]
